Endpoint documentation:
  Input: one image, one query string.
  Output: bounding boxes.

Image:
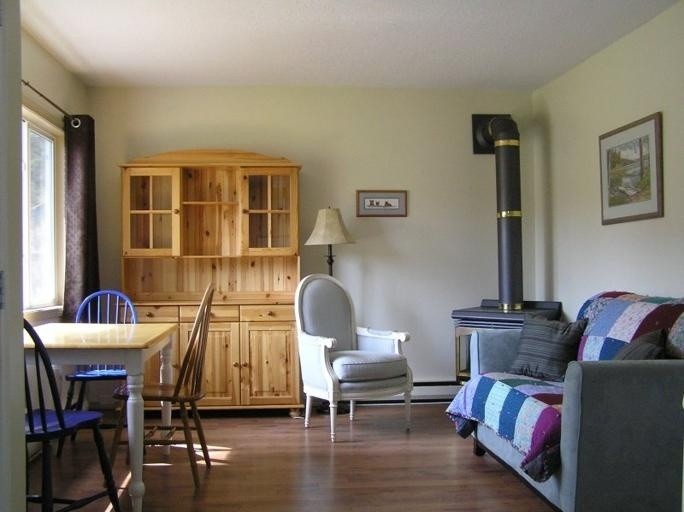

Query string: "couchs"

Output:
[444,290,682,511]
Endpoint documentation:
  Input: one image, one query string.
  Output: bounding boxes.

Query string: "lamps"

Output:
[304,205,354,276]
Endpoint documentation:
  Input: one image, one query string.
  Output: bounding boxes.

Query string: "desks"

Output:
[23,322,180,512]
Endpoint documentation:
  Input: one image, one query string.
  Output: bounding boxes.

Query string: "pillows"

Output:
[508,312,589,383]
[612,330,667,359]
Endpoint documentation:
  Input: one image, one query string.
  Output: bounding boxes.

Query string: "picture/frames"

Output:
[356,190,409,217]
[598,111,664,227]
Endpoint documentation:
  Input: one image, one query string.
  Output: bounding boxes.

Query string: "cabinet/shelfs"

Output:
[119,150,300,302]
[116,294,303,420]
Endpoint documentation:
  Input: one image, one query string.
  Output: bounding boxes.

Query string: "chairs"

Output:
[57,289,144,462]
[104,284,216,496]
[294,272,413,442]
[22,318,122,512]
